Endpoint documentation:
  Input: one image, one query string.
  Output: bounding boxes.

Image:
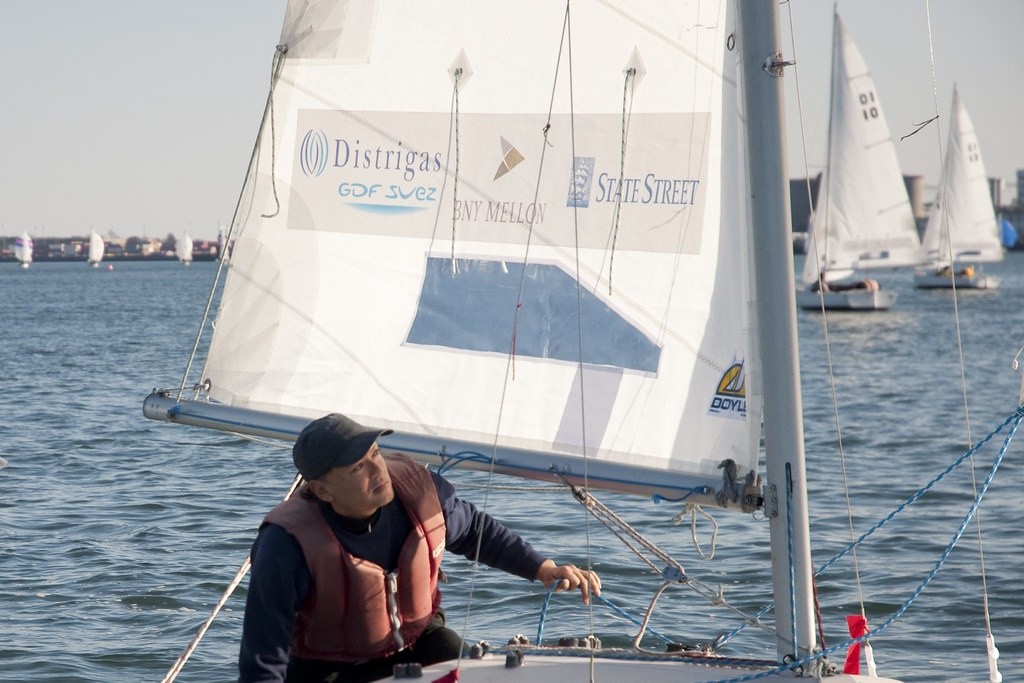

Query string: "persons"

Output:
[234,412,605,683]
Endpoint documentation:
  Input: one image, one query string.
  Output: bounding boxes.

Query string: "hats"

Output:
[292,412,395,483]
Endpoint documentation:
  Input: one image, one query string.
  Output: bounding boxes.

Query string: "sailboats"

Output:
[88,228,106,269]
[218,226,231,266]
[912,84,1006,289]
[177,229,194,266]
[795,1,924,309]
[12,231,34,269]
